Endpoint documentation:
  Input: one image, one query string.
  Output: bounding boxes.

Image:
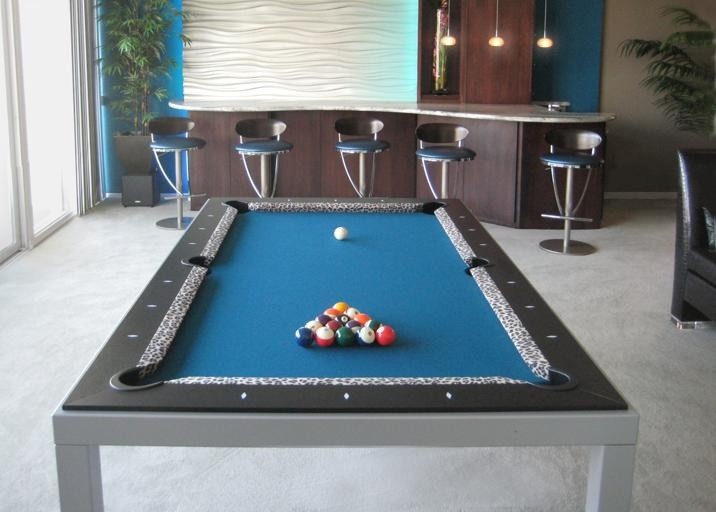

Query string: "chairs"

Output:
[145,115,205,229]
[332,116,389,197]
[233,118,291,197]
[537,127,604,255]
[661,148,715,331]
[416,122,476,199]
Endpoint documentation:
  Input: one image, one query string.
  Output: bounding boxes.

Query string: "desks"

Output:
[49,193,639,511]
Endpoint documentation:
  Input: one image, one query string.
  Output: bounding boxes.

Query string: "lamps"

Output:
[487,0,506,47]
[535,0,552,48]
[440,0,457,46]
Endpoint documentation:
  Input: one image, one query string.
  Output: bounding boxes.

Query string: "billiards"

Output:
[334,227,347,241]
[295,301,396,347]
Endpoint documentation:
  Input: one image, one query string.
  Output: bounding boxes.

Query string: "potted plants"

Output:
[87,1,192,208]
